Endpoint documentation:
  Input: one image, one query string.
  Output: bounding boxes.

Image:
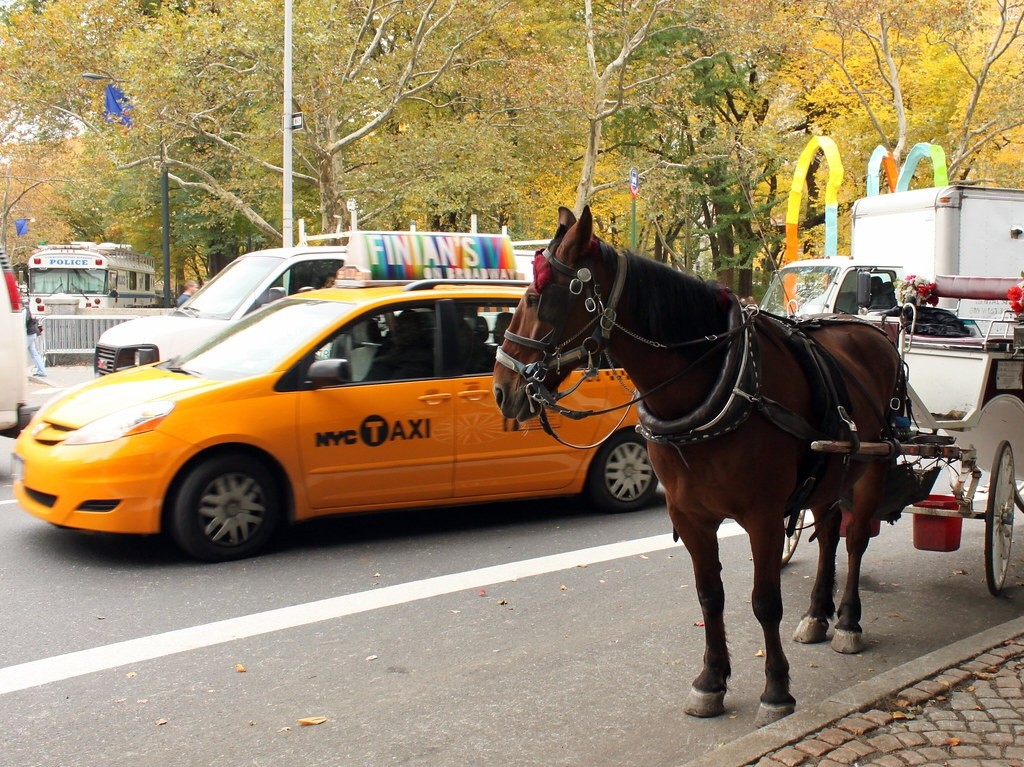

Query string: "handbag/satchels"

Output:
[26,308,38,335]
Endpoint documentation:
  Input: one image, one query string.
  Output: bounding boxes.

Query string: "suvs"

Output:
[83,206,557,379]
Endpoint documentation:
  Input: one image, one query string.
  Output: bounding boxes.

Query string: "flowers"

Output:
[893,275,939,308]
[1008,270,1024,317]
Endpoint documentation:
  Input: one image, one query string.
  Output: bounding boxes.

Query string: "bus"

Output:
[27,240,166,334]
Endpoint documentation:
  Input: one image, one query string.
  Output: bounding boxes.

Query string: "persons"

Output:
[307,309,432,384]
[25,307,47,377]
[177,280,197,307]
[492,312,513,344]
[323,272,336,288]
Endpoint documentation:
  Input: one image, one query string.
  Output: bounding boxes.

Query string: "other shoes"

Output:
[33,373,47,377]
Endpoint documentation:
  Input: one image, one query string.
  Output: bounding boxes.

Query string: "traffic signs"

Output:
[281,112,304,132]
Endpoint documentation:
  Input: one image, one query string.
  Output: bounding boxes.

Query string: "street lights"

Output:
[81,72,171,310]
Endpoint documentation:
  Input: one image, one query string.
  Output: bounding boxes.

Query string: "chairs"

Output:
[348,320,381,381]
[835,292,857,314]
[871,276,882,294]
[464,316,489,343]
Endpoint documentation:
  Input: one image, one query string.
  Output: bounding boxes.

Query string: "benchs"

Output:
[903,275,1023,350]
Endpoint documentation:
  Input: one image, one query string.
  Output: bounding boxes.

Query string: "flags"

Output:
[15,219,29,235]
[104,83,134,127]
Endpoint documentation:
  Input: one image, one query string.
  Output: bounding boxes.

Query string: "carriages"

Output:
[492,182,1024,729]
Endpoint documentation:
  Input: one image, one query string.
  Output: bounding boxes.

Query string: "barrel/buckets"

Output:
[911,493,963,552]
[839,508,881,537]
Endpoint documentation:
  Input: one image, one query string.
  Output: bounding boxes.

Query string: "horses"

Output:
[492,202,910,731]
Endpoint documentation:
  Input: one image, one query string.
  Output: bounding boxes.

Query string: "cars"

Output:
[10,221,661,563]
